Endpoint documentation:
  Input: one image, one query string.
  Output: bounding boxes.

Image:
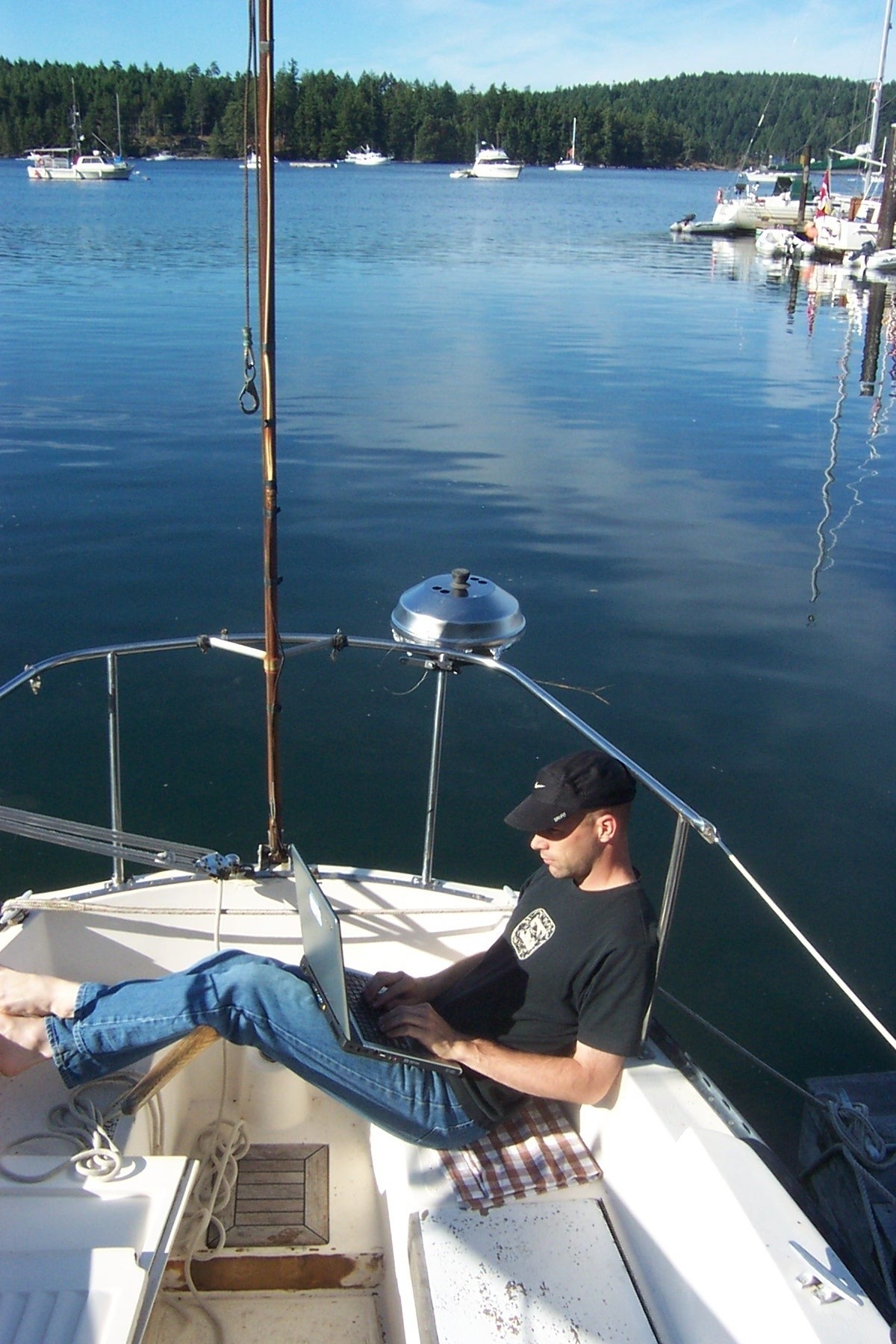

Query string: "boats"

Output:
[335,143,395,166]
[15,74,179,181]
[239,151,281,170]
[449,128,525,180]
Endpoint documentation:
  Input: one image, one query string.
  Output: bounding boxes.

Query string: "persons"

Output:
[0,751,658,1150]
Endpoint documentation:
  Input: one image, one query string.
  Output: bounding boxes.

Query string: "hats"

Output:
[503,751,639,831]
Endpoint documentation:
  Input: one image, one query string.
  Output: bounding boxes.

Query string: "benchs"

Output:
[8,1053,188,1157]
[373,1113,662,1344]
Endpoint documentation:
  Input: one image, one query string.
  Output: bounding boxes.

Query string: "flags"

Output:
[815,169,829,218]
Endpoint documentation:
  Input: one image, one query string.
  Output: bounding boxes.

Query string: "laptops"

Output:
[289,843,463,1075]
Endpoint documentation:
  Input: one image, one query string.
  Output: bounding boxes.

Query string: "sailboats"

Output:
[668,0,896,293]
[548,116,585,172]
